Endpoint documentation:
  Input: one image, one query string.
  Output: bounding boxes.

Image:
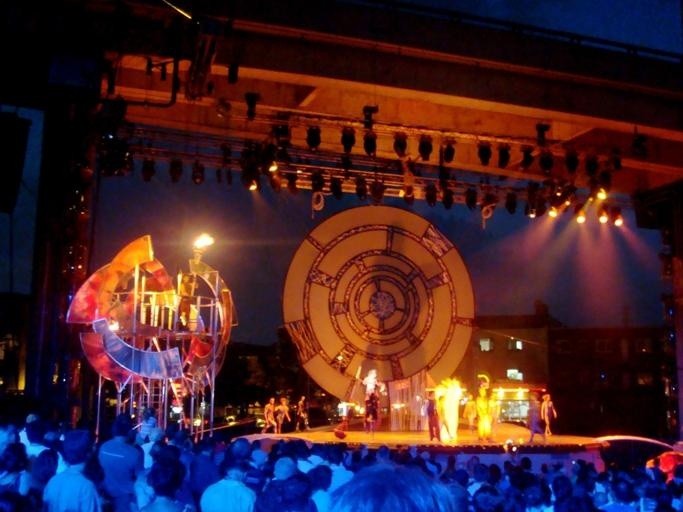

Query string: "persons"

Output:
[1,375,683,511]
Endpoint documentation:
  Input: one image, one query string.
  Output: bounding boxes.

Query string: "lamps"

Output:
[94,95,635,229]
